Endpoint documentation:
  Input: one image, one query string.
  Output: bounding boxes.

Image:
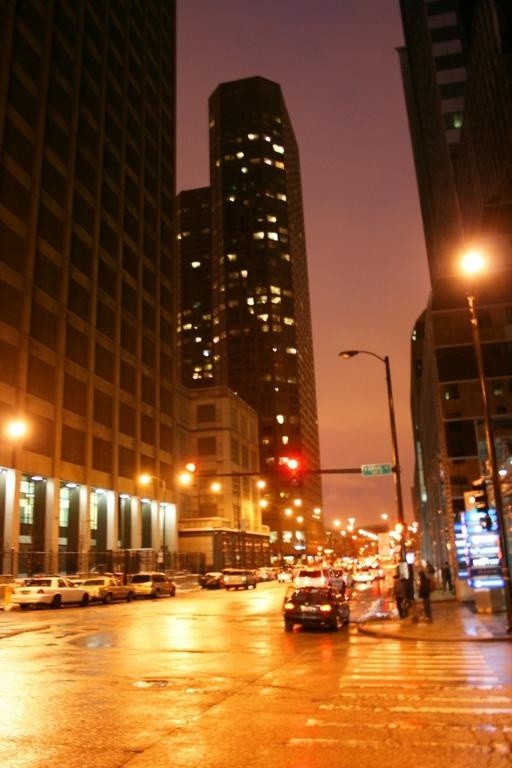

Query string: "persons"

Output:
[390,575,410,619]
[441,561,452,593]
[417,568,435,621]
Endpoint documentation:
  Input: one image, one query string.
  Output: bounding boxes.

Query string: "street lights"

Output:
[0,414,27,439]
[137,471,166,571]
[447,244,511,628]
[340,349,410,566]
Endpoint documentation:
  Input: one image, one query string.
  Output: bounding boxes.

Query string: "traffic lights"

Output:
[479,515,492,530]
[288,453,305,489]
[472,476,489,513]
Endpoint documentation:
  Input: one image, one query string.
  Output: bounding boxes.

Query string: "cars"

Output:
[195,543,389,633]
[13,568,177,607]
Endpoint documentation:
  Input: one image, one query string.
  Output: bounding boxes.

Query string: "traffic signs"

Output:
[361,462,392,476]
